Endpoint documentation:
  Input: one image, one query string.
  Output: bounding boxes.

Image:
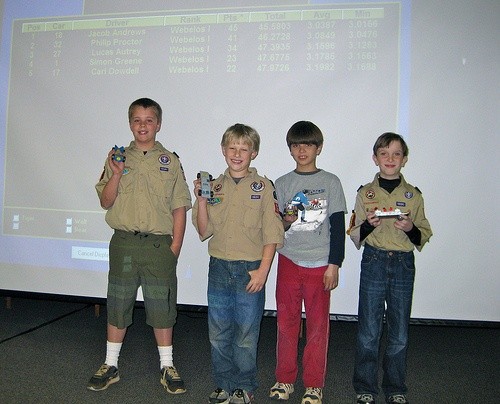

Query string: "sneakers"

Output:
[230,388,254,404]
[301,386,323,404]
[356,393,375,404]
[87,363,120,391]
[207,387,232,404]
[386,393,408,404]
[159,366,186,394]
[269,382,294,400]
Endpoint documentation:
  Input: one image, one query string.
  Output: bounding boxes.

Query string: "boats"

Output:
[374,211,409,217]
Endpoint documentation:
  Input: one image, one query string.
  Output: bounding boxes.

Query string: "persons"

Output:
[268,121,348,404]
[85,97,192,394]
[191,123,285,404]
[346,132,434,404]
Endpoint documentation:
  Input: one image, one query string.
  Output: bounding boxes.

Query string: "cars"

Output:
[197,171,214,198]
[112,145,126,162]
[283,204,298,217]
